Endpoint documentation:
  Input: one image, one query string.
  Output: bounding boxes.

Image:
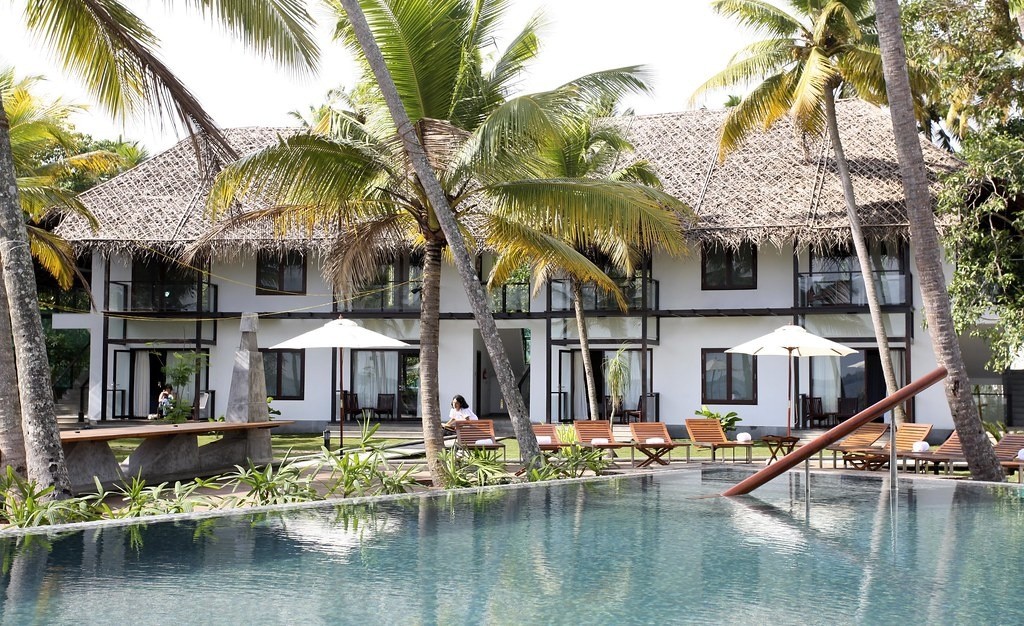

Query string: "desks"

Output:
[60,418,297,487]
[825,412,838,426]
[896,449,932,474]
[624,410,641,423]
[762,436,799,466]
[841,446,881,465]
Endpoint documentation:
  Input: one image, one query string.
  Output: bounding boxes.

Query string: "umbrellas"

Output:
[724,323,860,454]
[268,314,421,461]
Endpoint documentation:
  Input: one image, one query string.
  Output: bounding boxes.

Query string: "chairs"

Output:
[948,432,1024,480]
[635,395,642,422]
[630,420,691,466]
[362,394,394,419]
[574,419,633,465]
[451,419,506,469]
[878,424,933,471]
[157,393,177,418]
[835,397,859,425]
[514,424,571,478]
[686,418,754,465]
[900,430,967,476]
[605,394,626,423]
[343,393,362,421]
[825,421,889,469]
[806,396,826,427]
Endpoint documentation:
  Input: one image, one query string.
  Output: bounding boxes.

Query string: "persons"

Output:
[157,382,174,419]
[441,395,479,435]
